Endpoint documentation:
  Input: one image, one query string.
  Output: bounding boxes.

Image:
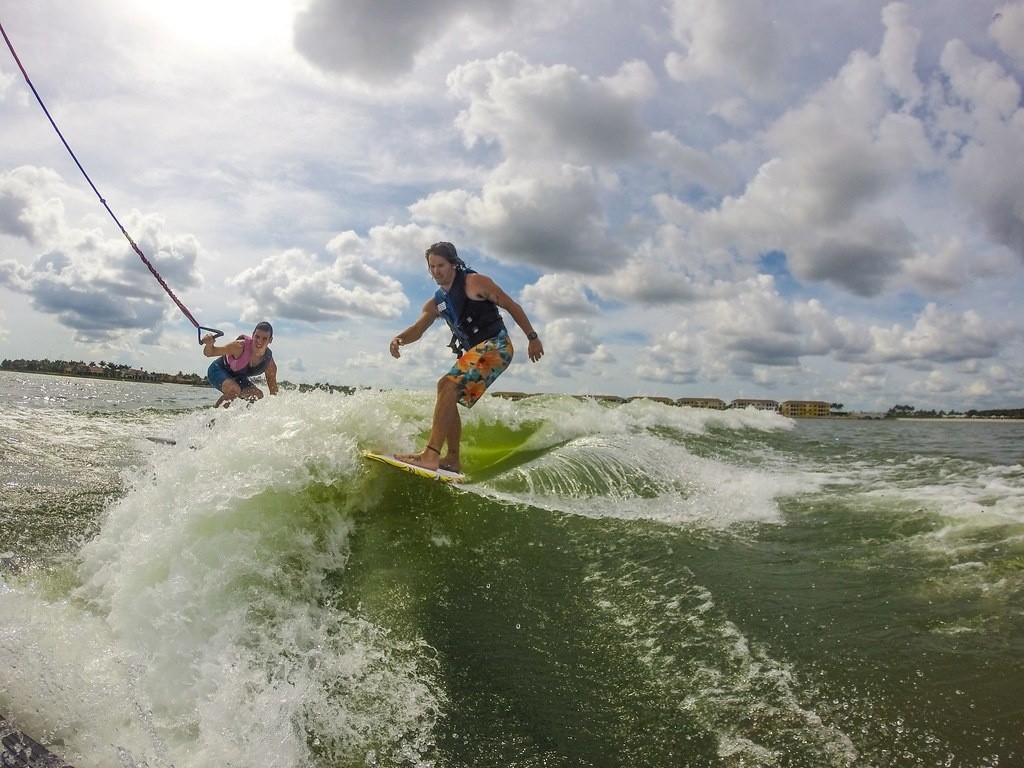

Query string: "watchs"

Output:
[527,332,537,340]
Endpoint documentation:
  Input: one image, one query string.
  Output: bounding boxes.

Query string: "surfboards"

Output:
[357,450,468,486]
[146,434,204,452]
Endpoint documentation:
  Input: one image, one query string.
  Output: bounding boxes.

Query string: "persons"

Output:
[202,321,278,408]
[390,242,545,471]
